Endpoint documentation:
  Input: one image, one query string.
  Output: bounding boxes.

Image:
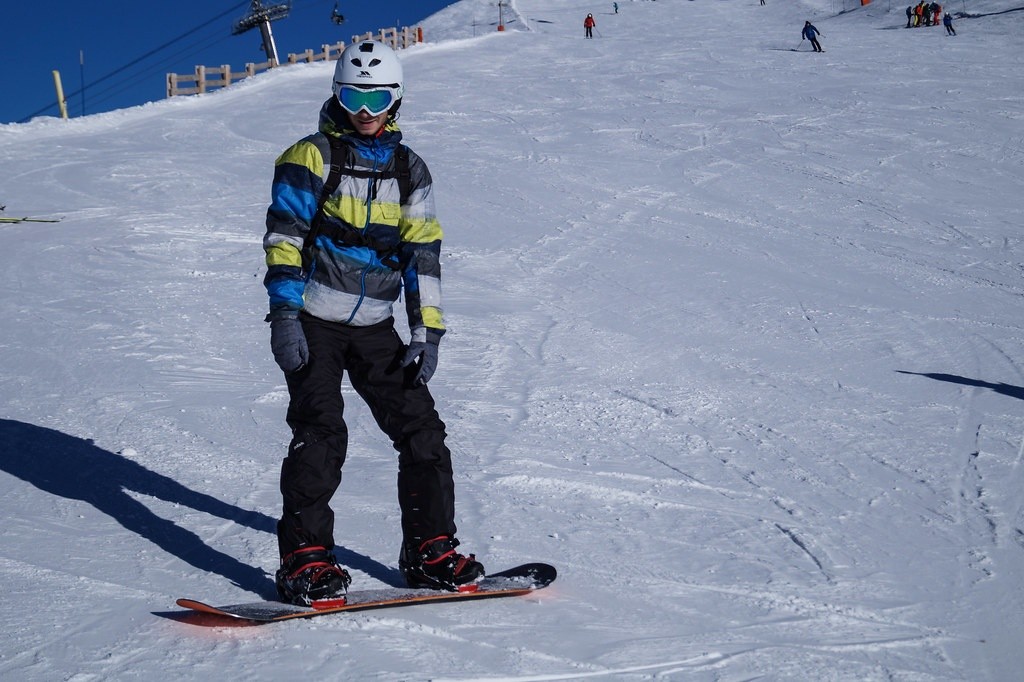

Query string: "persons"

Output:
[584,13,595,39]
[943,12,956,36]
[418,27,423,42]
[802,21,822,53]
[333,9,344,24]
[906,0,942,27]
[264,40,486,603]
[613,2,618,13]
[761,0,765,5]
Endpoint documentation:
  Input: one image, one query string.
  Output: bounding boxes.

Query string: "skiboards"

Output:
[0,215,67,224]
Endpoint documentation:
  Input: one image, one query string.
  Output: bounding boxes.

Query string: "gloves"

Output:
[264,301,310,375]
[399,326,447,388]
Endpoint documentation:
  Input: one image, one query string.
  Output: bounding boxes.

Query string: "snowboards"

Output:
[175,563,556,622]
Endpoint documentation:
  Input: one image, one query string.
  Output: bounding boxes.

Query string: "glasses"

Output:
[337,84,396,118]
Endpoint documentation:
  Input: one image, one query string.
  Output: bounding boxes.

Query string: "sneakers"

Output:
[398,541,485,585]
[276,547,342,600]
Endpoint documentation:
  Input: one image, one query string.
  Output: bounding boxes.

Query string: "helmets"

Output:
[331,40,405,100]
[944,12,948,15]
